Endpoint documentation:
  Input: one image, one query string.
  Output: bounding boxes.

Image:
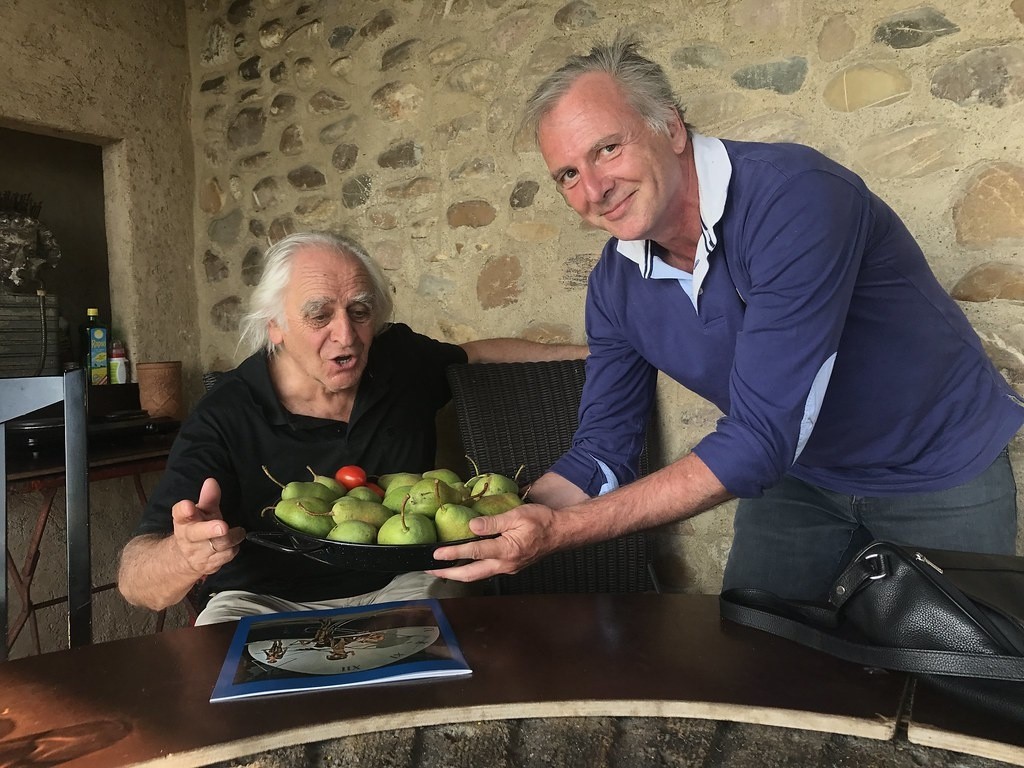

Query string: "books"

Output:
[209,599,475,703]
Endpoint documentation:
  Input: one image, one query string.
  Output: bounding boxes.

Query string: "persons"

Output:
[428,49,1023,594]
[117,232,591,627]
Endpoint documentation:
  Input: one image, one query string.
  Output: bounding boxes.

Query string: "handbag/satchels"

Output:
[718,540,1024,726]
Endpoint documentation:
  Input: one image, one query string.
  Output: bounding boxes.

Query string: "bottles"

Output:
[83,307,108,385]
[109,340,126,384]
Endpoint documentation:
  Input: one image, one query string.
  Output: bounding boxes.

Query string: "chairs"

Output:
[453,359,673,595]
[187,370,438,632]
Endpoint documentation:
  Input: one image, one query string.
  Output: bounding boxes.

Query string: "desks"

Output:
[8,445,171,654]
[0,594,1024,768]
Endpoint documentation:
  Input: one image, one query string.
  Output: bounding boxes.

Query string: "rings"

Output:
[208,539,219,553]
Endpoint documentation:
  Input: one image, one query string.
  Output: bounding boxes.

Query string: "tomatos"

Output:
[336,466,366,487]
[366,482,384,496]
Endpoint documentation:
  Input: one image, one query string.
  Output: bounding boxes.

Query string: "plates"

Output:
[270,494,529,563]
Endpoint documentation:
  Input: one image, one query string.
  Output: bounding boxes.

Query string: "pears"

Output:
[260,456,532,545]
